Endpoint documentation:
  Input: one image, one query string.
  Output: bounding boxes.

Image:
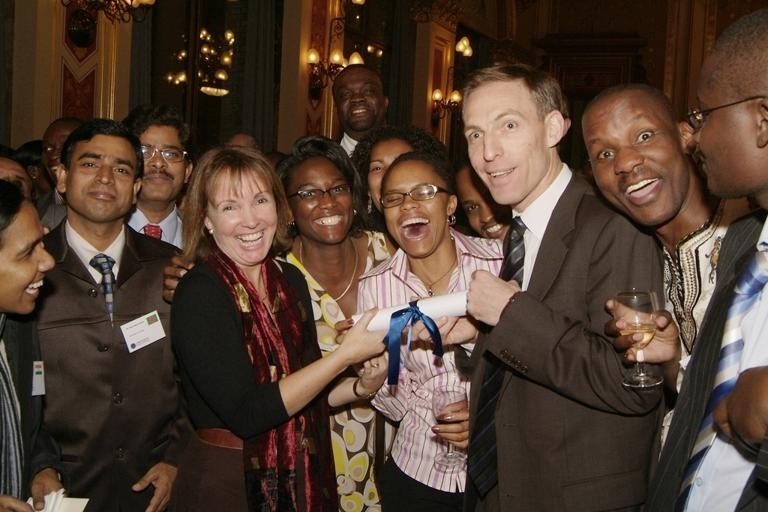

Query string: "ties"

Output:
[89,253,116,317]
[674,249,768,512]
[144,224,162,240]
[465,216,527,499]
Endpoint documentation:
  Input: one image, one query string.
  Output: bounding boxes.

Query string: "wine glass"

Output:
[613,287,668,393]
[432,388,469,470]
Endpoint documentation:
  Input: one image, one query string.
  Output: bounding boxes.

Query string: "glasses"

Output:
[689,96,767,128]
[380,184,453,208]
[141,145,192,163]
[287,184,352,199]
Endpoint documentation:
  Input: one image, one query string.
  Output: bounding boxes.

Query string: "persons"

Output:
[642,6,767,512]
[456,61,668,511]
[1,101,512,512]
[331,63,391,158]
[580,83,757,436]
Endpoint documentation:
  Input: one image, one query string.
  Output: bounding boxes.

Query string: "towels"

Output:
[307,16,366,101]
[61,0,156,48]
[432,62,473,128]
[200,28,235,97]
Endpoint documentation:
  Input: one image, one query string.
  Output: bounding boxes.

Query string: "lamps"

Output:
[166,34,188,86]
